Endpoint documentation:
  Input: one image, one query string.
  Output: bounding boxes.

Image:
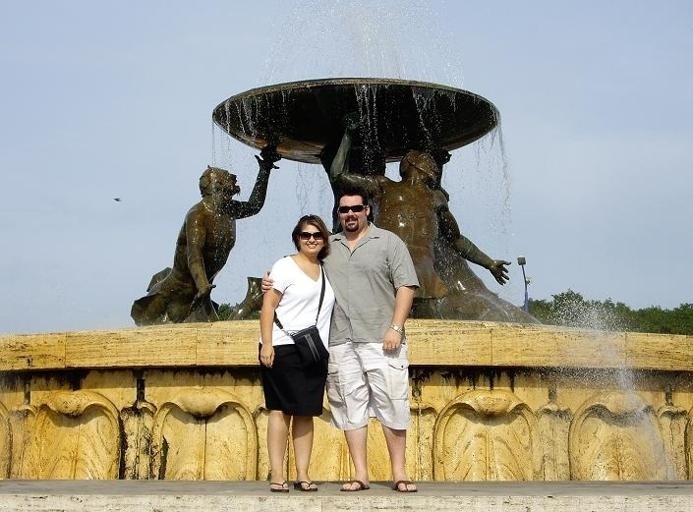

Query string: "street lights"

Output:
[517,255,531,311]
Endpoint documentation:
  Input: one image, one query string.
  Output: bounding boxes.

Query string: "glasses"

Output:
[298,232,323,239]
[338,205,367,212]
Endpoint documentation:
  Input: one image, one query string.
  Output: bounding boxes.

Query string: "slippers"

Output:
[294,482,318,491]
[340,482,371,491]
[392,481,417,493]
[270,481,290,492]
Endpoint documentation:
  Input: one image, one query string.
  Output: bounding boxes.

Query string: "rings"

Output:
[391,347,395,349]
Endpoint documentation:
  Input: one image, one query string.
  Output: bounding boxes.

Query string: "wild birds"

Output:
[114,198,121,202]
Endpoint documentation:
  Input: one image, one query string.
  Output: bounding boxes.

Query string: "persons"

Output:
[256,214,337,493]
[122,141,281,324]
[259,185,417,494]
[326,110,512,318]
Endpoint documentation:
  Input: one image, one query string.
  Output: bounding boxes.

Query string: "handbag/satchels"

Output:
[291,326,328,366]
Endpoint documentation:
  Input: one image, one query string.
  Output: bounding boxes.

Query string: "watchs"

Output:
[388,323,404,336]
